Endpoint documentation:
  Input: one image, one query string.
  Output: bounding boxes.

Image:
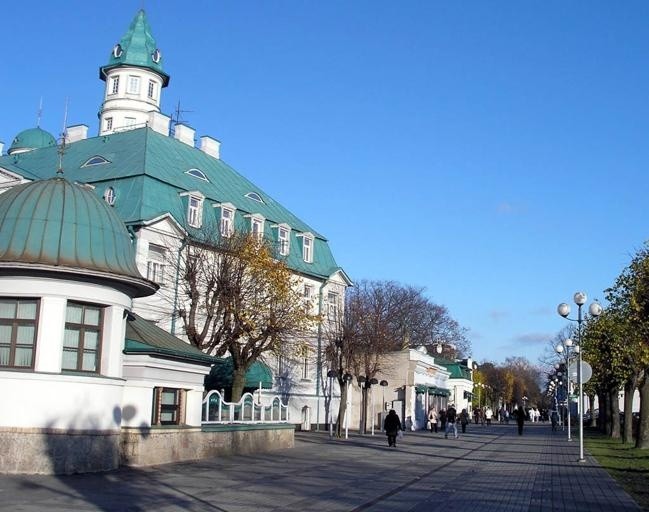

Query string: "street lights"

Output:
[545,359,566,433]
[522,396,528,405]
[484,385,491,406]
[558,292,602,463]
[555,340,579,441]
[476,382,485,410]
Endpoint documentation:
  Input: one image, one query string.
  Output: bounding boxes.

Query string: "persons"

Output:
[428,404,541,439]
[384,410,404,447]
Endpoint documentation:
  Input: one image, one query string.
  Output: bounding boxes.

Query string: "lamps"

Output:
[123,309,136,321]
